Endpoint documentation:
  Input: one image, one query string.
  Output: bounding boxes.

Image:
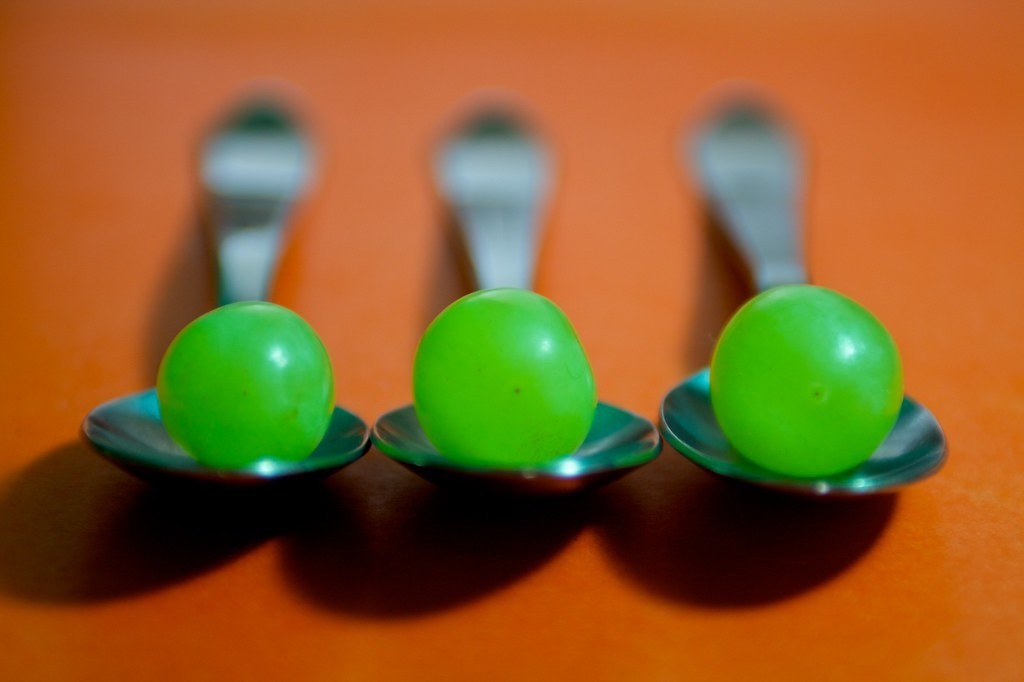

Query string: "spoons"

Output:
[661,107,947,489]
[375,112,659,485]
[81,101,373,481]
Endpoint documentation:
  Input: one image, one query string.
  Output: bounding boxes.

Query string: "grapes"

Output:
[156,299,336,466]
[411,284,597,465]
[711,285,902,471]
